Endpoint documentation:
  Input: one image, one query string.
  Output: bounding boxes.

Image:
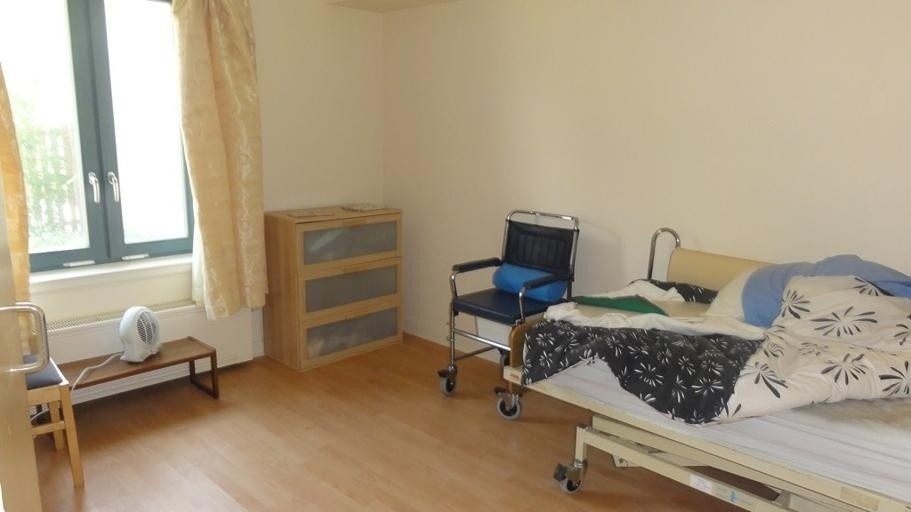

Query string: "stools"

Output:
[22,353,83,488]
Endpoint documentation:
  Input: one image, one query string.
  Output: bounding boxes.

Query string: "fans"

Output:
[117,306,160,363]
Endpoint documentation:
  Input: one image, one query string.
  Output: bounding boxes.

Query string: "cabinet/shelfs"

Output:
[262,203,402,374]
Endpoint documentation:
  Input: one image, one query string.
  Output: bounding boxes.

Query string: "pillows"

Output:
[703,255,909,330]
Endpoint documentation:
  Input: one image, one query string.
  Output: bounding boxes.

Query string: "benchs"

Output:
[57,336,217,440]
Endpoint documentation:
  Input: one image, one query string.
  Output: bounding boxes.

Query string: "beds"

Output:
[500,230,911,511]
